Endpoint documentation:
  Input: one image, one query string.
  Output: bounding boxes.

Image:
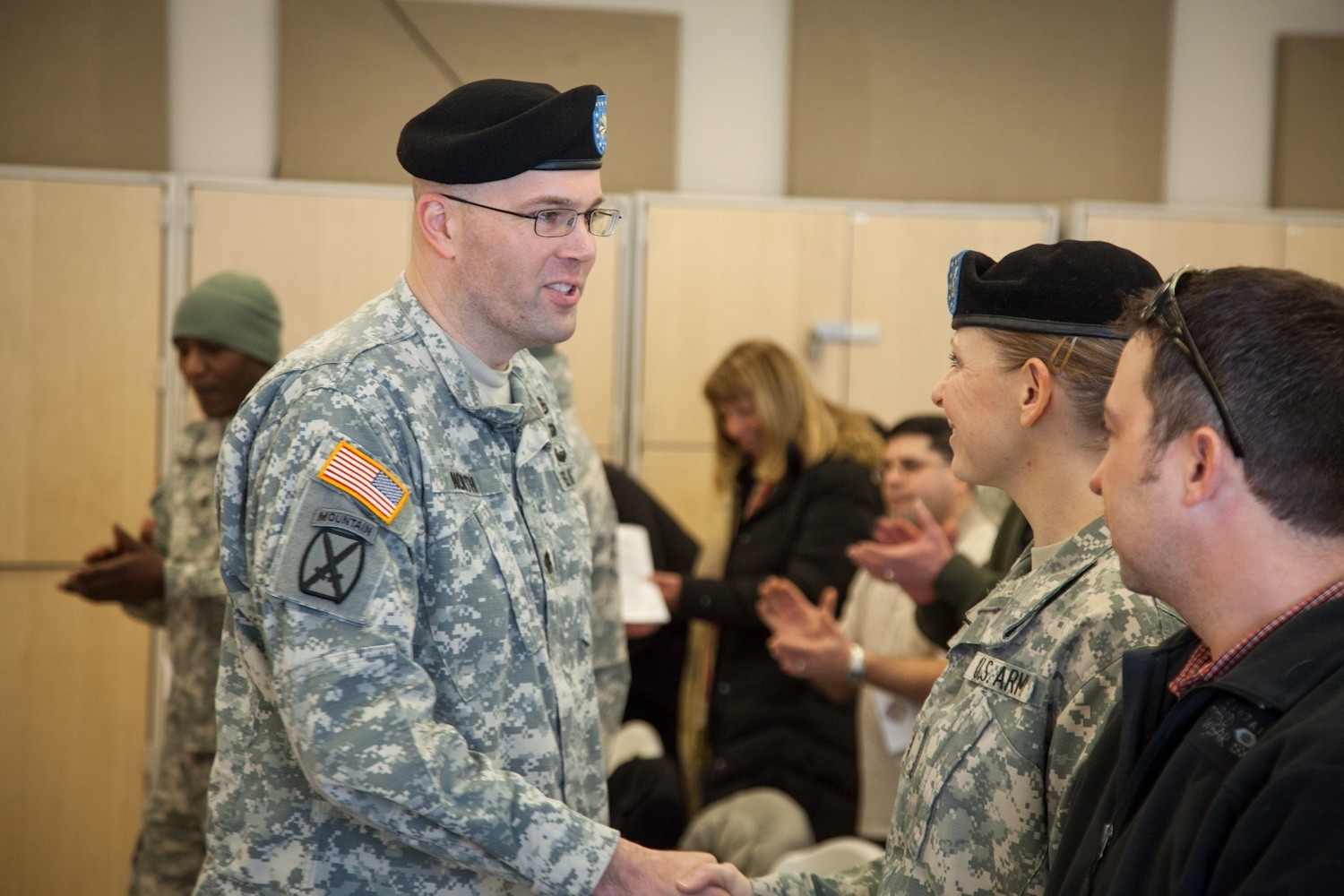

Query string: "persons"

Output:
[190,78,731,896]
[60,240,1343,896]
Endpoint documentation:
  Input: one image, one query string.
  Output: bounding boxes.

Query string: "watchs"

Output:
[846,642,870,693]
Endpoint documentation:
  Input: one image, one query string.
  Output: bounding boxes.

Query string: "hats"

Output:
[396,79,607,185]
[947,240,1163,339]
[172,271,281,365]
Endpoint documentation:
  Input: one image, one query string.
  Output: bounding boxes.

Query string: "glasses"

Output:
[441,192,622,238]
[1142,264,1246,459]
